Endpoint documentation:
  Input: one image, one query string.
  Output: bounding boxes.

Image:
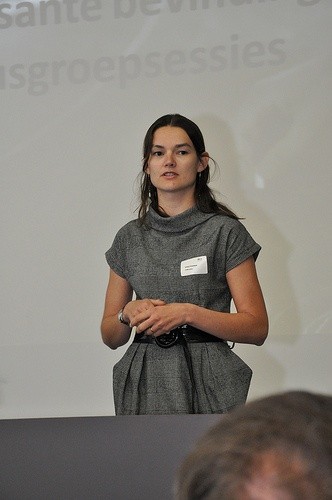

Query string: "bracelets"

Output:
[118,306,130,325]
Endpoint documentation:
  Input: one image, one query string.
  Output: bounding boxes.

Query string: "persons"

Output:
[172,388,332,500]
[99,112,270,417]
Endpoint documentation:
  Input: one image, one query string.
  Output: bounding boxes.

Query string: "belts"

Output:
[133,327,226,349]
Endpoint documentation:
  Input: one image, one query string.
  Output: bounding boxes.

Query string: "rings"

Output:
[150,326,154,333]
